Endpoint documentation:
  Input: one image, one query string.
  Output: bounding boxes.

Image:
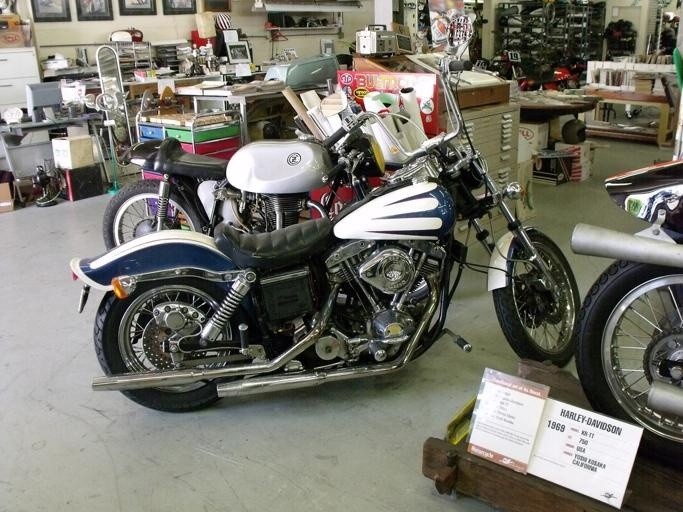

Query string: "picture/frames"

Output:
[31,0,231,22]
[224,40,252,64]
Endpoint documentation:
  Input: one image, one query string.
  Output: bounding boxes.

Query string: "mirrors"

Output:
[96,45,138,188]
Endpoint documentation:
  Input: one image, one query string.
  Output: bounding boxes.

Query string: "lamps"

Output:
[193,12,216,72]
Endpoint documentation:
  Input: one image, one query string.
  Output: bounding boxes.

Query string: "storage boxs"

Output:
[51,135,103,202]
[0,178,16,213]
[532,140,594,186]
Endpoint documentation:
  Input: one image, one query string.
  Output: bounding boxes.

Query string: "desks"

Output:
[121,77,203,117]
[1,113,112,208]
[175,84,328,145]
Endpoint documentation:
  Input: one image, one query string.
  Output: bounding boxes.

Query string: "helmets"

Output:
[664,14,680,22]
[538,63,554,80]
[562,120,586,144]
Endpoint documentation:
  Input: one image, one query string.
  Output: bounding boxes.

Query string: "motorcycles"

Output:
[571,158,682,467]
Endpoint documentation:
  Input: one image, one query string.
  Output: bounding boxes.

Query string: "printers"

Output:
[264,53,339,90]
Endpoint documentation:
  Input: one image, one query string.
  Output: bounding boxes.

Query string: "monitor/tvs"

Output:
[396,33,412,53]
[25,81,63,122]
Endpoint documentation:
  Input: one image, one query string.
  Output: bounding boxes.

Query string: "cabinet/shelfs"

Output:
[493,0,606,83]
[134,105,245,230]
[115,41,183,81]
[0,46,44,171]
[437,102,519,248]
